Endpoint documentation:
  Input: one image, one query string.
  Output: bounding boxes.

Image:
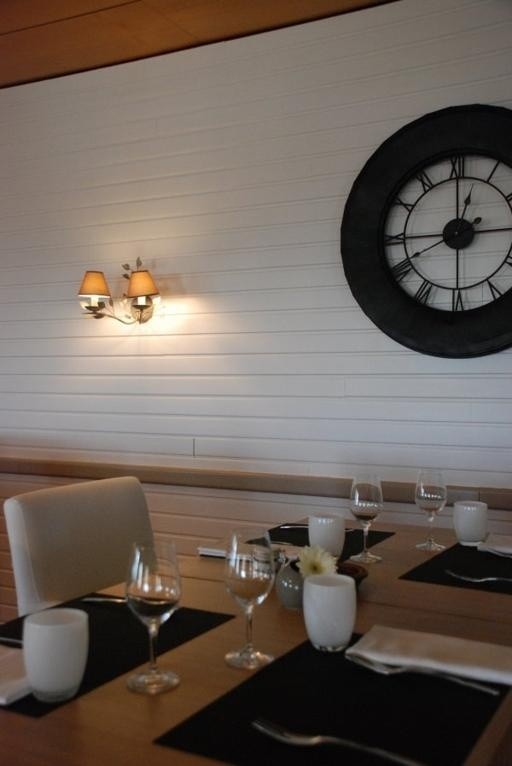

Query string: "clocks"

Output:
[340,104,510,359]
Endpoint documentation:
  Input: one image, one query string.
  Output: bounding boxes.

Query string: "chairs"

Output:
[1,476,158,617]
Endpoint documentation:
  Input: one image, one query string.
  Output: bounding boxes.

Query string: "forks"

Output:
[249,716,421,765]
[345,652,502,698]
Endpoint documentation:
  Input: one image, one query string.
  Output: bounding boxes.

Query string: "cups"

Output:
[306,512,347,563]
[21,606,90,703]
[452,499,490,547]
[301,572,358,655]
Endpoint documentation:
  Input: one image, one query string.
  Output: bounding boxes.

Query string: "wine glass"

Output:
[349,472,386,565]
[414,466,450,554]
[222,526,277,672]
[122,536,184,698]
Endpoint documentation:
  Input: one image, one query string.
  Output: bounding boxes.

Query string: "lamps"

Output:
[78,256,157,325]
